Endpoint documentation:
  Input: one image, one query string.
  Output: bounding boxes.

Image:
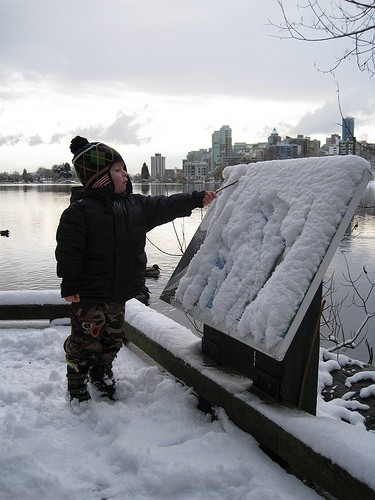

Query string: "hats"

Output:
[69,135,134,195]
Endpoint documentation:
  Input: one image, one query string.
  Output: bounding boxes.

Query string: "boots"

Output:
[91,364,116,401]
[66,371,92,403]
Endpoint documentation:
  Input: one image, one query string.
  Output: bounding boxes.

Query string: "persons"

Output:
[54,137,219,412]
